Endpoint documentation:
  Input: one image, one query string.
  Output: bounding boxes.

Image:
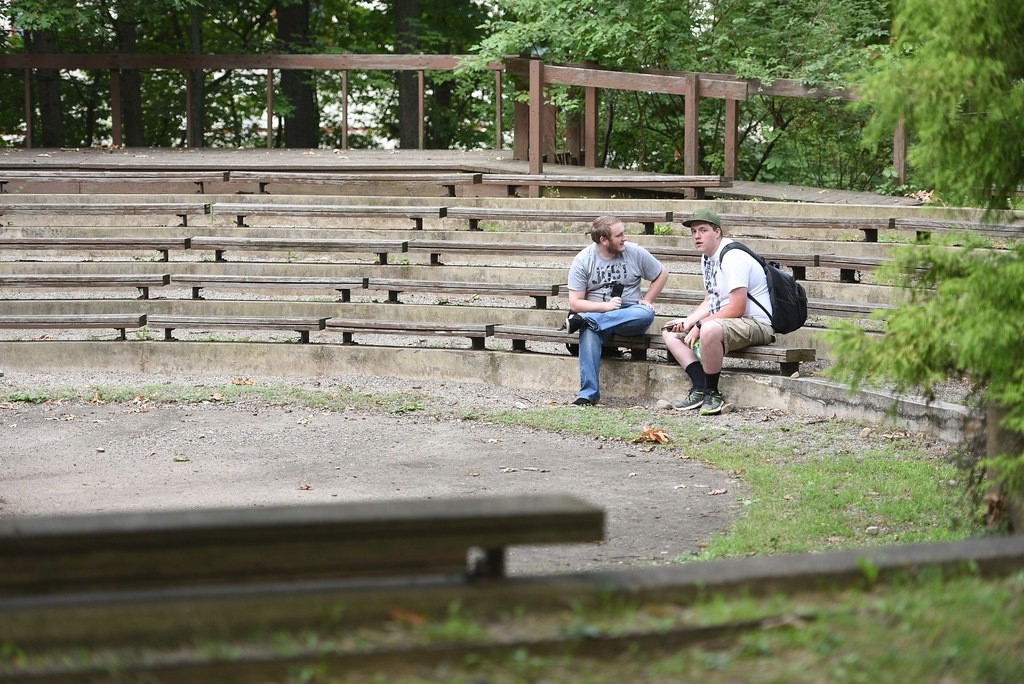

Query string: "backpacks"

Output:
[719,242,807,335]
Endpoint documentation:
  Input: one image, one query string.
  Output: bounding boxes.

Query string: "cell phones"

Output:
[661,321,685,331]
[610,282,623,297]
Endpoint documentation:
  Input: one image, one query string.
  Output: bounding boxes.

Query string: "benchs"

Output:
[0,170,1023,377]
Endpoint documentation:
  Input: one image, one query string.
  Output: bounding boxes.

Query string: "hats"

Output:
[682,209,721,228]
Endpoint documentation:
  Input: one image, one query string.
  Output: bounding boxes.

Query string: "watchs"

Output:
[696,320,702,329]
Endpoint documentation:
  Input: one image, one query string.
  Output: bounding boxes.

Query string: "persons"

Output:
[565,216,668,408]
[661,208,777,417]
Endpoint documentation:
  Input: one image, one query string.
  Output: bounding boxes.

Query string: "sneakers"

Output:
[569,398,594,408]
[673,387,722,411]
[565,311,588,334]
[699,388,725,415]
